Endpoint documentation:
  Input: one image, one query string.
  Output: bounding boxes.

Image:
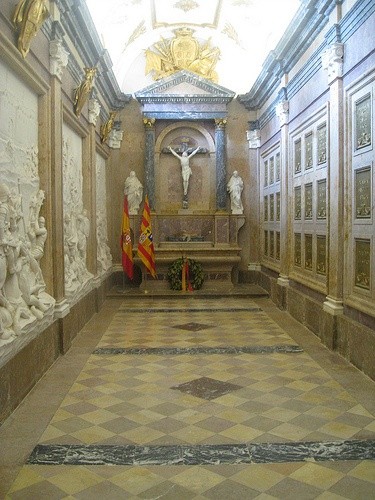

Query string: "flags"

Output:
[138,192,157,278]
[120,191,136,281]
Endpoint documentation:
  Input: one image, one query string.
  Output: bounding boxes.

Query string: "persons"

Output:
[227,169,244,215]
[0,181,119,352]
[168,145,201,195]
[123,171,144,215]
